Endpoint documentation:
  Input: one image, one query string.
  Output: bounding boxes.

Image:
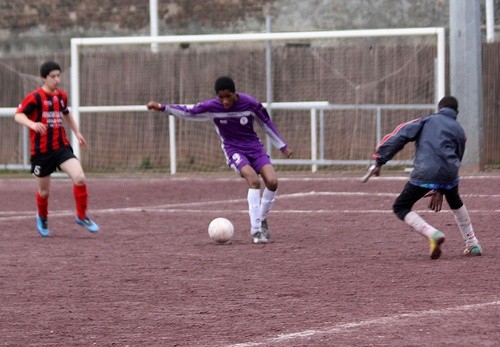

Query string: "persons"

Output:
[360,96,483,259]
[13,60,100,237]
[147,76,294,244]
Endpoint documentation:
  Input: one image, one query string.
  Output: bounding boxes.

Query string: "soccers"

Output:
[208,217,234,242]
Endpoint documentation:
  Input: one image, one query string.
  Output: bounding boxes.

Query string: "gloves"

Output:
[423,191,443,212]
[361,164,380,183]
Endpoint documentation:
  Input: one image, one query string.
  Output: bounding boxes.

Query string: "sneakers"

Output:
[35,210,49,236]
[261,219,270,239]
[430,231,446,259]
[74,215,100,233]
[462,246,482,256]
[252,233,268,243]
